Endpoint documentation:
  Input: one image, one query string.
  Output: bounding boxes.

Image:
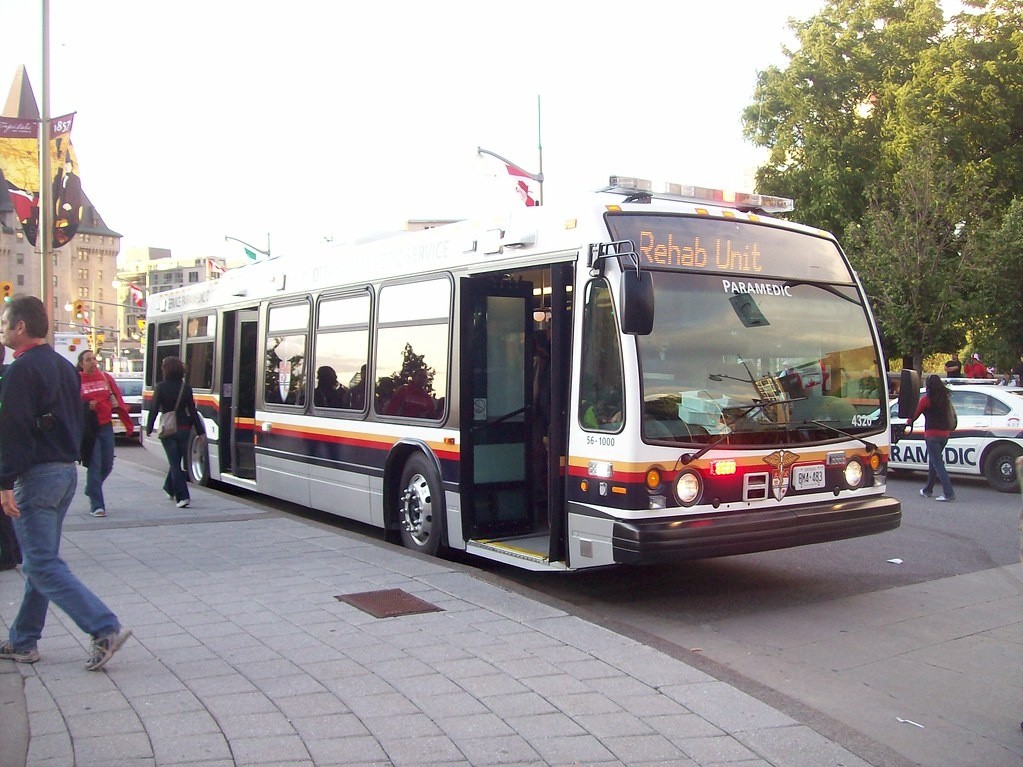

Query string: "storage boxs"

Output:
[676,403,723,426]
[679,388,732,413]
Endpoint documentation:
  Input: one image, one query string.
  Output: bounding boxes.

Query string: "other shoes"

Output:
[90,508,107,517]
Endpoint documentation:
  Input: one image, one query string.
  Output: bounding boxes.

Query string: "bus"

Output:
[139,175,921,573]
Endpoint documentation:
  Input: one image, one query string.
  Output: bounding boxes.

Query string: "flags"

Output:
[210,260,225,273]
[130,284,142,307]
[505,166,538,207]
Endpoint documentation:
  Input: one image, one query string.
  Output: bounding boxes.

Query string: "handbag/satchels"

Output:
[382,385,404,415]
[157,411,176,439]
[101,372,119,409]
[947,399,957,430]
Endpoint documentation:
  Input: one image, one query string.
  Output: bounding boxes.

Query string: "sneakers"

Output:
[0,640,40,663]
[175,499,190,508]
[920,489,928,498]
[163,486,174,500]
[935,495,954,502]
[81,625,132,671]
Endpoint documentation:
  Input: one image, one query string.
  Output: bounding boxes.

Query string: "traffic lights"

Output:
[73,300,85,322]
[0,281,13,305]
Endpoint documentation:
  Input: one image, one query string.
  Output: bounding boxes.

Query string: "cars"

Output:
[109,378,144,435]
[864,385,1023,493]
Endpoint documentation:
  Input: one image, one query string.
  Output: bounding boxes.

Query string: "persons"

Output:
[76,350,133,518]
[905,374,957,501]
[474,316,554,501]
[964,353,987,378]
[0,294,132,673]
[1012,356,1023,387]
[146,356,204,507]
[778,360,789,378]
[945,353,961,377]
[997,370,1017,387]
[315,365,444,419]
[987,365,994,376]
